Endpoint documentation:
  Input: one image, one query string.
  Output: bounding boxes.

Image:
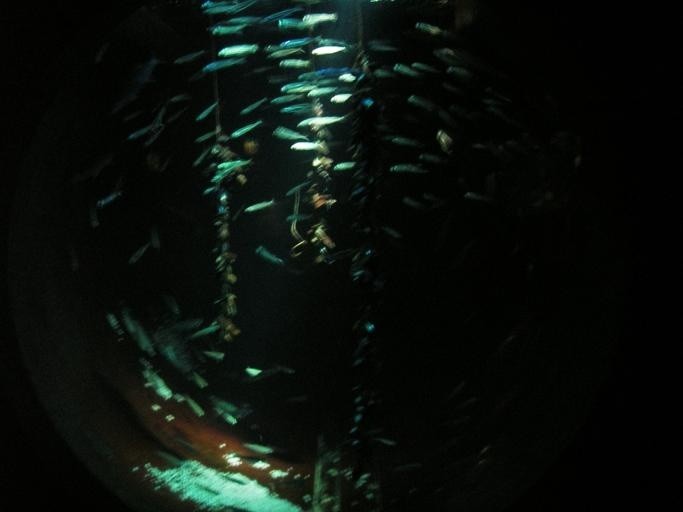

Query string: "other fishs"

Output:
[75,1,492,511]
